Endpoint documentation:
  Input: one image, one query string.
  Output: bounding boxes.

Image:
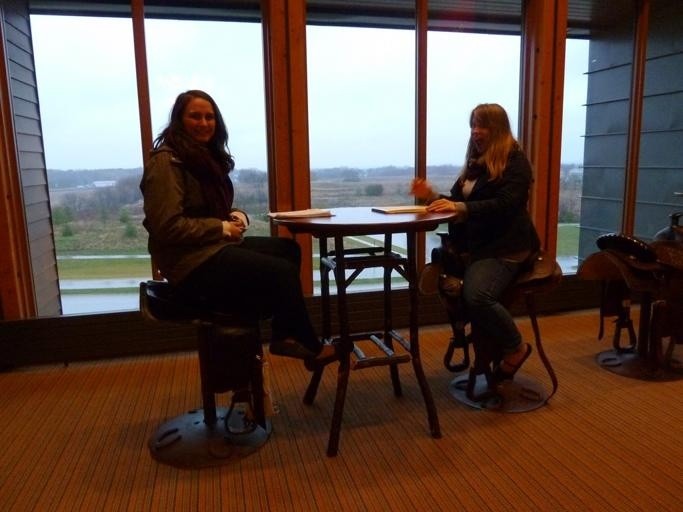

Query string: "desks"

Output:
[272,205,460,459]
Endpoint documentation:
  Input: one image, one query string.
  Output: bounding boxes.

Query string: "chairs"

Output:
[139,280,274,470]
[578,233,683,382]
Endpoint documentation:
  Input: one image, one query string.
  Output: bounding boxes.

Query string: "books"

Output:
[372,206,429,213]
[266,208,333,220]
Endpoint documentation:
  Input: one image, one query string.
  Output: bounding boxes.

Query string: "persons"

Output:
[140,98,333,362]
[407,102,541,383]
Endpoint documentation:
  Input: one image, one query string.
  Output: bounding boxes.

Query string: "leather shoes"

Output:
[497,342,531,378]
[303,337,355,371]
[270,336,302,361]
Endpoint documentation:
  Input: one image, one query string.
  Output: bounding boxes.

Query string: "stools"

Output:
[430,247,563,413]
